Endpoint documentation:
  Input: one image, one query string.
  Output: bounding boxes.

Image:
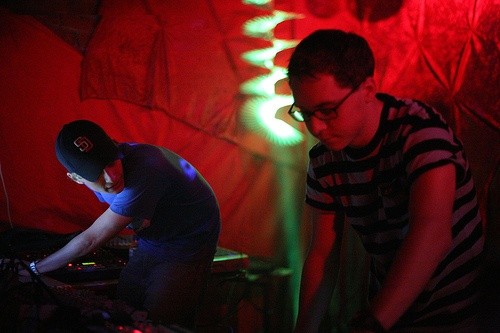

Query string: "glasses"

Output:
[288,79,363,122]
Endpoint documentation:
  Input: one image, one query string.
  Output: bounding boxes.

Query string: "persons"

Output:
[284,27,485,333]
[14,119,221,328]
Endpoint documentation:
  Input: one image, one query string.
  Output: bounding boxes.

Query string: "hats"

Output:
[54,120,122,182]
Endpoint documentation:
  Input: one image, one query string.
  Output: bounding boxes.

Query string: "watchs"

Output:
[29,260,40,277]
[349,308,387,333]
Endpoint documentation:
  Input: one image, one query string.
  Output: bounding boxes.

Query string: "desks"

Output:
[51,242,250,333]
